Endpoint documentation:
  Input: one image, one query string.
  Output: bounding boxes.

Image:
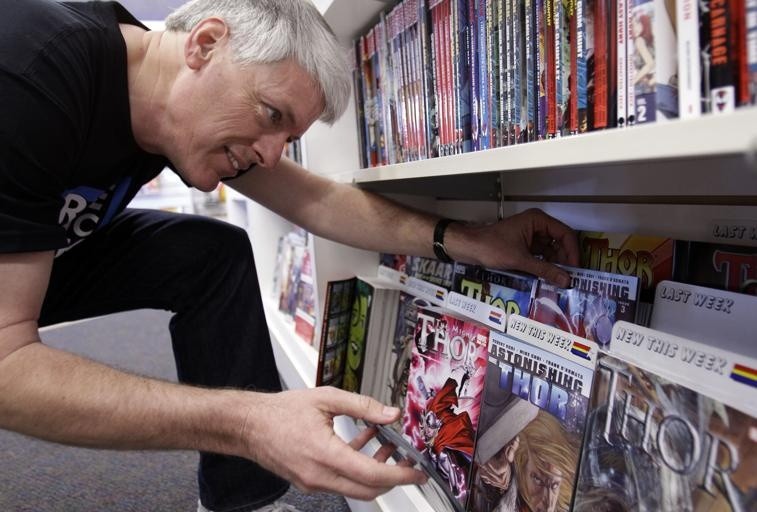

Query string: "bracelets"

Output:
[432,217,454,264]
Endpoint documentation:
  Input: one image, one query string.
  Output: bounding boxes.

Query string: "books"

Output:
[314,260,575,512]
[575,228,757,511]
[355,1,573,169]
[574,0,755,140]
[268,228,319,352]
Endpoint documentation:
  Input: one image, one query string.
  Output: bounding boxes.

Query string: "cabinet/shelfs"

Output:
[224,136,316,382]
[293,1,757,511]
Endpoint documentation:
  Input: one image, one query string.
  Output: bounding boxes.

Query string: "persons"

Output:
[474,405,584,511]
[626,10,656,93]
[418,378,476,507]
[342,276,372,396]
[0,0,574,511]
